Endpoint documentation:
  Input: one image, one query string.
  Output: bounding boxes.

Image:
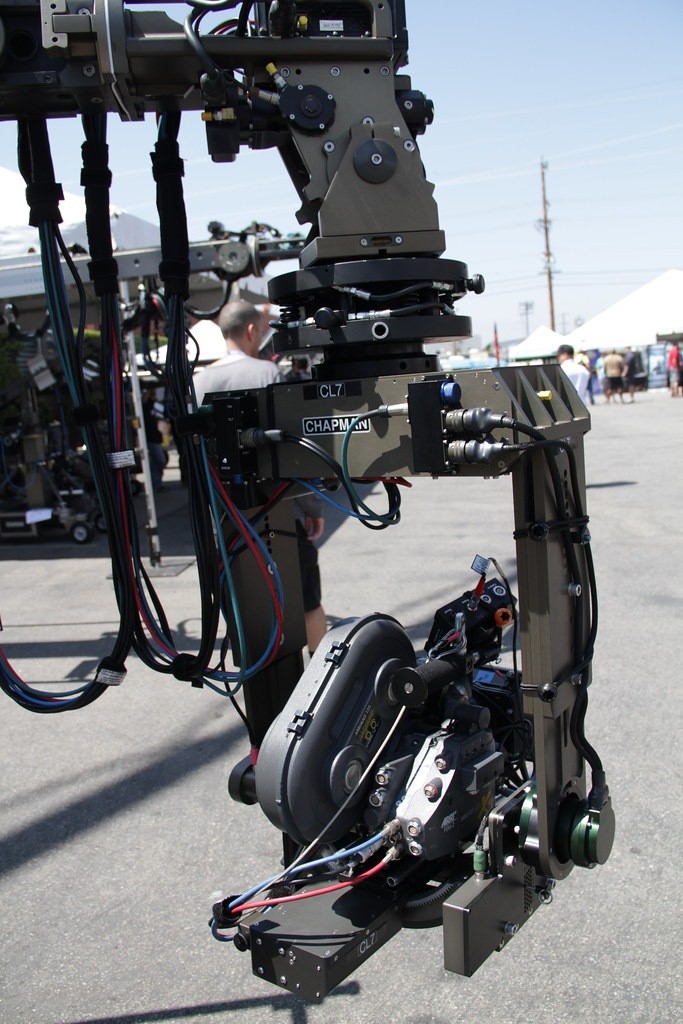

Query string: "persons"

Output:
[553,344,590,403]
[575,346,639,404]
[668,341,680,397]
[192,303,330,666]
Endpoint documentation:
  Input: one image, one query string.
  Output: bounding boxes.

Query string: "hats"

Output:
[553,345,574,355]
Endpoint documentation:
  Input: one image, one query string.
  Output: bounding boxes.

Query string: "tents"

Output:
[565,268,683,350]
[506,325,587,367]
